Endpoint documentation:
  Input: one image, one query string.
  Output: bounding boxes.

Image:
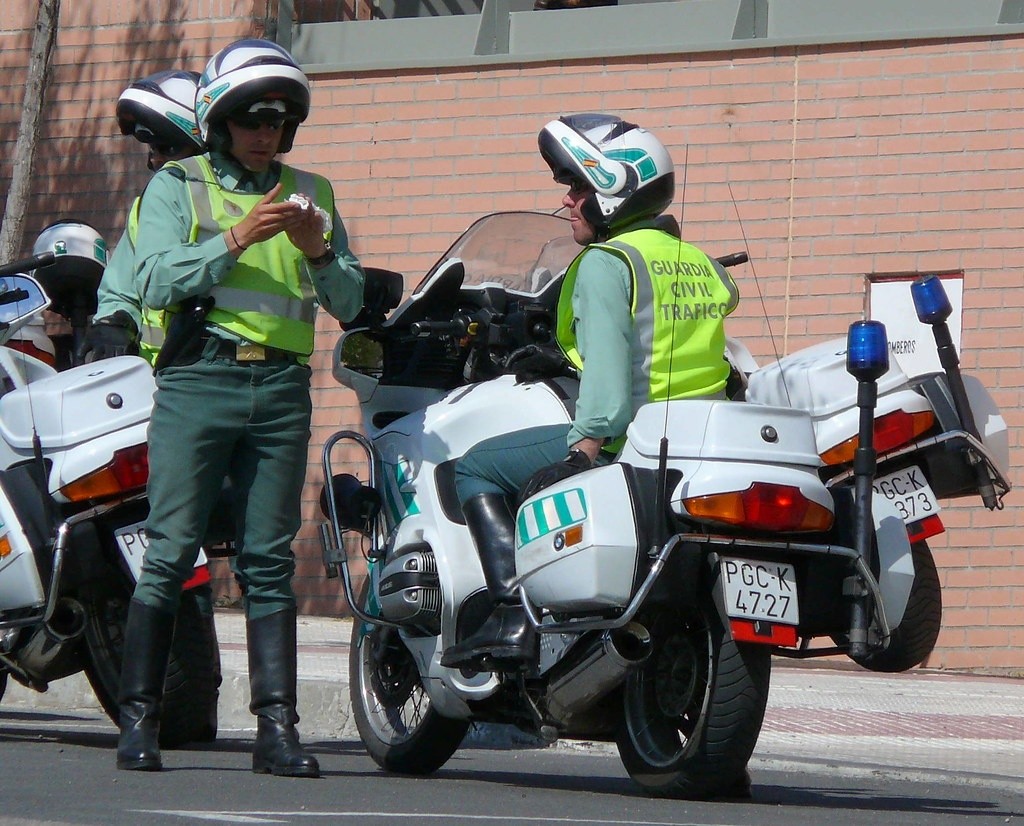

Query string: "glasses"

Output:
[145,142,182,155]
[233,115,286,130]
[570,179,589,195]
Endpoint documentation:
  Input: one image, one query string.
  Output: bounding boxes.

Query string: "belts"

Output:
[198,341,289,362]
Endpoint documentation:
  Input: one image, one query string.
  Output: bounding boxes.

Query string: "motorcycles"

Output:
[320,211,1015,797]
[0,218,227,749]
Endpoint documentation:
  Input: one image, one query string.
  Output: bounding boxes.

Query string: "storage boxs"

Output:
[904,369,1006,499]
[613,401,837,535]
[510,461,692,615]
[2,355,160,502]
[751,333,935,468]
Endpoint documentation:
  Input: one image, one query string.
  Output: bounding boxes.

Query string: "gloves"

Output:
[76,309,142,361]
[506,343,566,382]
[517,448,591,507]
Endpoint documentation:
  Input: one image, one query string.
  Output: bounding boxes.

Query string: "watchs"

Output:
[303,239,335,266]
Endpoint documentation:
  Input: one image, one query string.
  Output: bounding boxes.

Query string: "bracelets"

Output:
[230,227,247,251]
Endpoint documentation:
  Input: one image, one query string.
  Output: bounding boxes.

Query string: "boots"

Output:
[117,597,176,768]
[246,607,319,775]
[441,492,539,671]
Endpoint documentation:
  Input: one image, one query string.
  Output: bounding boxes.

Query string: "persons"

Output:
[79,70,208,367]
[115,37,365,777]
[439,113,740,669]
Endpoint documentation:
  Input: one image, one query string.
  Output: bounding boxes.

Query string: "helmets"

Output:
[34,219,109,308]
[539,113,675,231]
[117,70,205,152]
[194,39,311,154]
